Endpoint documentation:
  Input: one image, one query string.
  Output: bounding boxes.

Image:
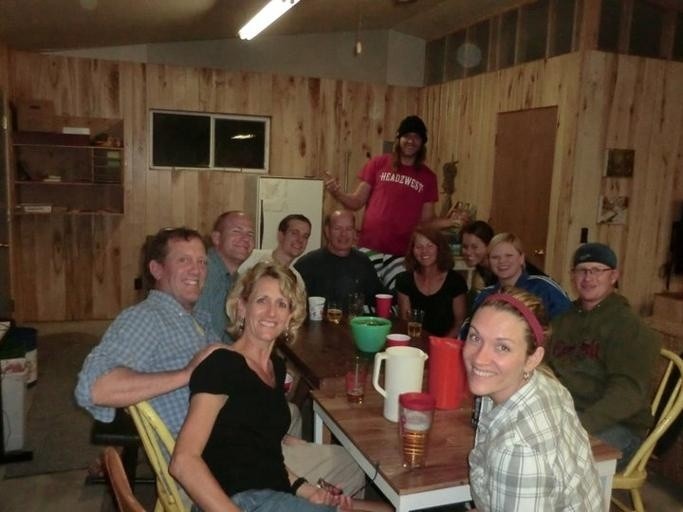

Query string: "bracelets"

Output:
[290,477,308,496]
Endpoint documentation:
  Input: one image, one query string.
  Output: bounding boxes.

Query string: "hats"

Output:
[397,116,428,142]
[574,243,620,291]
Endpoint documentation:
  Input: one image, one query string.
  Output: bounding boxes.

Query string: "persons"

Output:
[460,220,553,295]
[325,115,470,288]
[458,234,571,340]
[546,243,662,474]
[462,286,602,512]
[239,213,313,299]
[74,228,366,500]
[294,210,389,317]
[395,226,469,339]
[168,262,395,512]
[192,210,255,345]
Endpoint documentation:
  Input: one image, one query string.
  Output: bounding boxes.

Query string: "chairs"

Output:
[105,446,157,512]
[125,400,197,512]
[609,350,683,512]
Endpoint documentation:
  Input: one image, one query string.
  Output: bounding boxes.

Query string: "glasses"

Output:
[316,463,379,500]
[573,267,610,277]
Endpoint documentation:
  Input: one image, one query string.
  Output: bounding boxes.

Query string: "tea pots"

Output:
[427,335,470,410]
[372,347,428,423]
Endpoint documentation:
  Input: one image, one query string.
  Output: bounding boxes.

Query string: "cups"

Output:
[347,291,364,328]
[399,394,437,472]
[407,309,424,337]
[375,294,393,320]
[345,357,369,407]
[327,304,344,322]
[308,296,325,320]
[386,334,411,347]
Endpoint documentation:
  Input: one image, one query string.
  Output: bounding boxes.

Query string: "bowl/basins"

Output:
[351,317,392,352]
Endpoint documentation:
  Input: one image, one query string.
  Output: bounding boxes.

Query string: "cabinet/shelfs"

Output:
[13,110,127,217]
